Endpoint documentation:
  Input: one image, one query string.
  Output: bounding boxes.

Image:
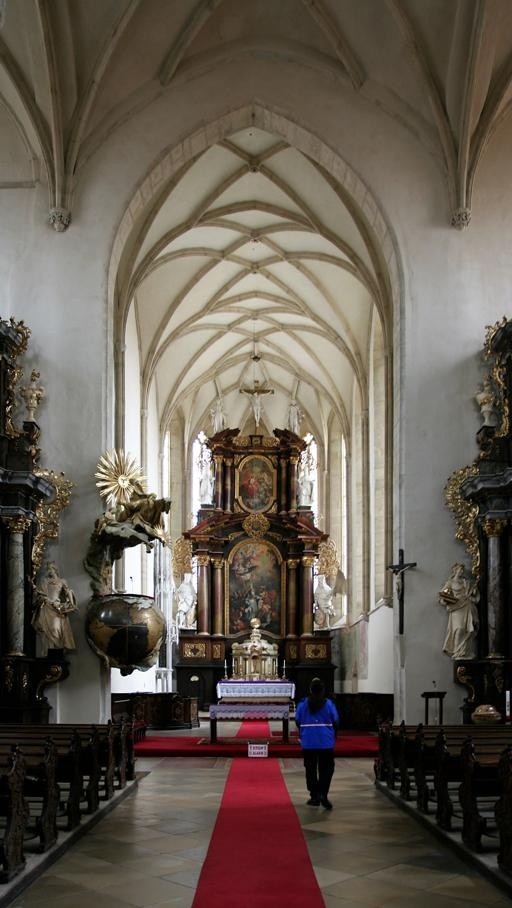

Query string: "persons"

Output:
[294,676,340,811]
[115,491,158,520]
[241,389,272,427]
[230,543,281,634]
[314,575,335,632]
[243,465,270,500]
[437,561,480,660]
[31,558,78,659]
[173,571,196,629]
[286,399,302,435]
[210,399,225,433]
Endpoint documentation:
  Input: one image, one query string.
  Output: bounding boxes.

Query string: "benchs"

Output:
[373,721,512,877]
[0,713,138,885]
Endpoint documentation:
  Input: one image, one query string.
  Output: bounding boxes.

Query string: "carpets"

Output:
[191,756,326,908]
[133,720,380,752]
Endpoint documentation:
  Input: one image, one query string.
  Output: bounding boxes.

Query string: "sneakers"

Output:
[307,799,320,806]
[320,794,333,810]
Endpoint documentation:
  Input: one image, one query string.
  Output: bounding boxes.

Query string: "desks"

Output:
[209,704,289,744]
